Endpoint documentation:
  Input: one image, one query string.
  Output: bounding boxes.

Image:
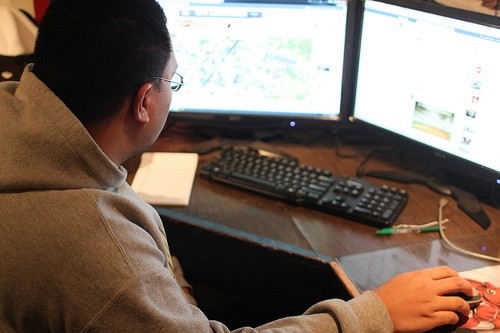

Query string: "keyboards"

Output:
[199,143,409,230]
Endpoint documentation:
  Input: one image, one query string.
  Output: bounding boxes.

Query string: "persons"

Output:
[0,0,478,333]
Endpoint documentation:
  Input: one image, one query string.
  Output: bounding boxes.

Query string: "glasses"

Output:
[148,72,183,91]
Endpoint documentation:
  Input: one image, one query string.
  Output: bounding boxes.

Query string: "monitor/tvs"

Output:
[155,0,356,131]
[347,0,500,195]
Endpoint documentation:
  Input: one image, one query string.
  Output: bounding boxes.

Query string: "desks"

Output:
[123,121,500,297]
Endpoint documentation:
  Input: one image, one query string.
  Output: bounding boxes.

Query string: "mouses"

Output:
[442,292,482,315]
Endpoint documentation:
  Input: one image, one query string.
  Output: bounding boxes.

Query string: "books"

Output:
[131,151,199,207]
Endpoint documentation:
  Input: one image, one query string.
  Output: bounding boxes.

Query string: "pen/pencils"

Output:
[375,226,447,235]
